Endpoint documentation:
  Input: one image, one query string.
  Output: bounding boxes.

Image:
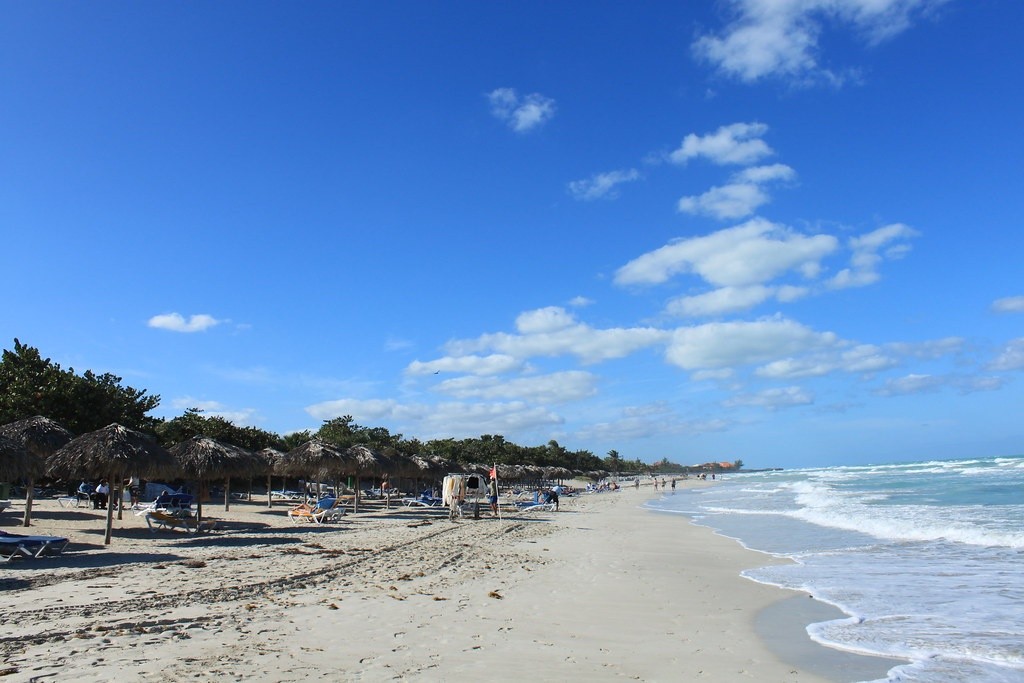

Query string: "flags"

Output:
[490,469,495,477]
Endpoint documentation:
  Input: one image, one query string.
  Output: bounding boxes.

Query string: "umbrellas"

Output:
[247,448,290,507]
[45,423,185,545]
[0,415,73,526]
[344,444,575,510]
[145,434,248,531]
[275,440,356,502]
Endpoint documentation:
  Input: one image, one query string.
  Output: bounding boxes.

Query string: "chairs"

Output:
[513,492,558,512]
[132,492,217,534]
[265,487,397,525]
[59,490,87,509]
[402,497,442,508]
[0,530,69,563]
[76,488,92,509]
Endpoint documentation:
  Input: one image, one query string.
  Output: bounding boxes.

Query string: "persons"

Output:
[661,478,666,490]
[293,503,316,512]
[697,473,715,480]
[490,478,500,516]
[587,482,618,493]
[671,478,676,489]
[96,479,124,510]
[634,477,639,487]
[127,476,139,508]
[654,478,658,490]
[544,486,562,511]
[136,490,167,517]
[79,478,107,510]
